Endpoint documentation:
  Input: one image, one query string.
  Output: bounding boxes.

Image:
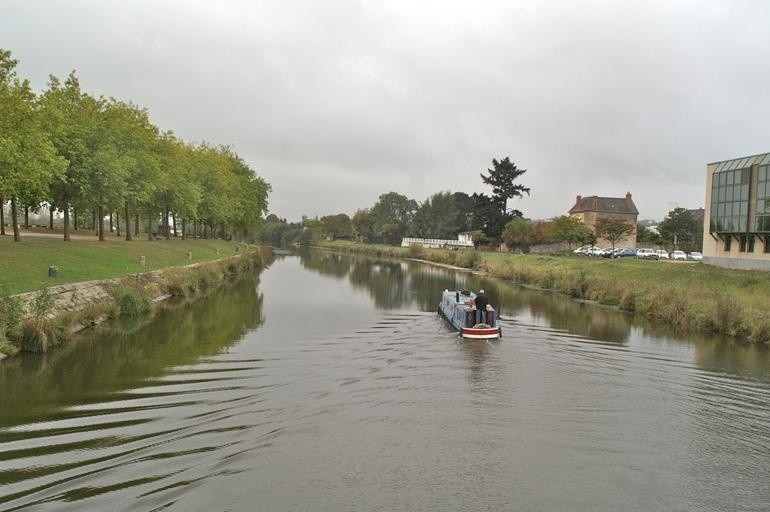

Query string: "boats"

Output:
[437,286,503,340]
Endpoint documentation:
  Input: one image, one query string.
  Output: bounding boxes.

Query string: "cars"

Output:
[572,244,670,260]
[688,251,703,261]
[670,250,687,260]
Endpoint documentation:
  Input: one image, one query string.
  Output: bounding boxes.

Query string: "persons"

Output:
[473,289,489,324]
